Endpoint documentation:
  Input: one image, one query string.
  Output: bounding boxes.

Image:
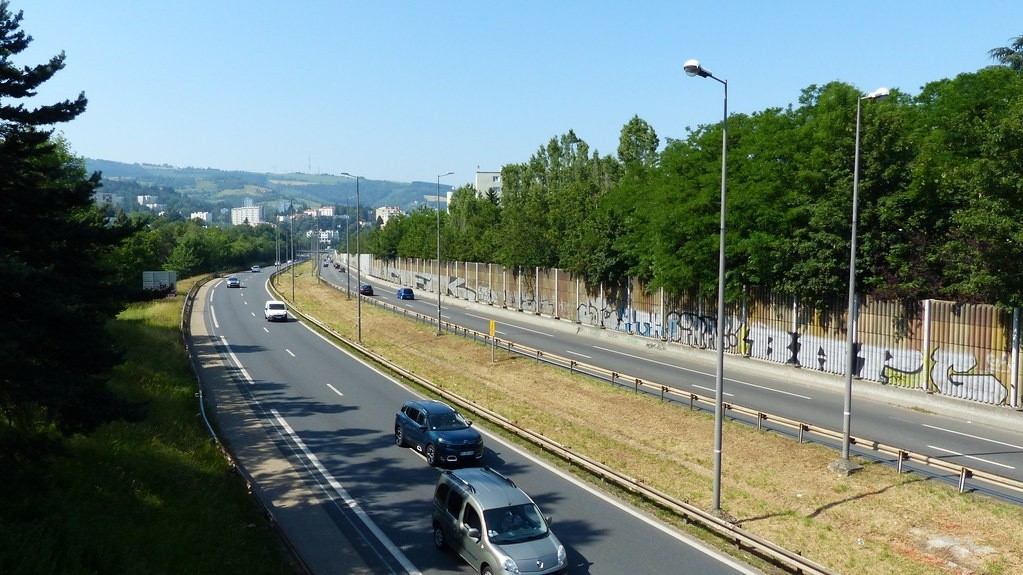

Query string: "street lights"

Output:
[339,172,361,342]
[683,57,729,510]
[346,195,357,298]
[842,86,890,459]
[436,171,455,330]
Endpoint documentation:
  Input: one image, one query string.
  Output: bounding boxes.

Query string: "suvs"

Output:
[431,466,568,575]
[395,400,484,467]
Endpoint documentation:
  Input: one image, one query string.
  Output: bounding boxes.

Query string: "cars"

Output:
[252,266,260,273]
[397,287,414,300]
[264,300,289,322]
[323,256,345,272]
[356,284,373,296]
[227,277,240,288]
[275,261,281,267]
